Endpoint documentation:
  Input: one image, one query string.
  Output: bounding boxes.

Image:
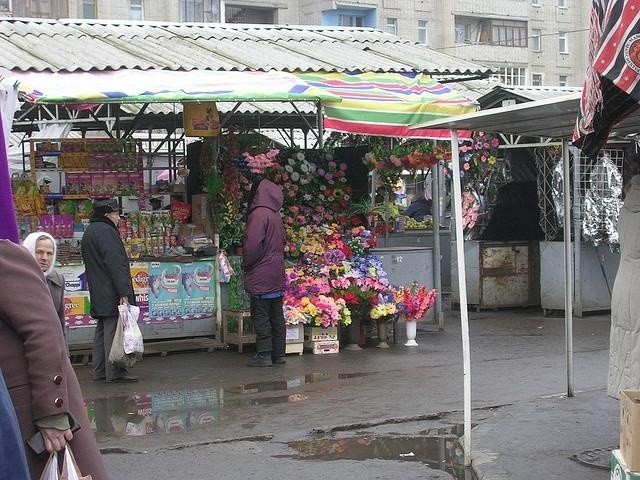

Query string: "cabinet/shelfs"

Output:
[30,137,145,201]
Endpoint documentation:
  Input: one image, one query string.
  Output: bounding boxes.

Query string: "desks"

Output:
[449,239,540,313]
[223,308,256,353]
[539,241,621,318]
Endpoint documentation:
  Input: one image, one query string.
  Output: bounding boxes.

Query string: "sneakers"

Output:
[248,353,272,367]
[106,375,138,382]
[273,355,286,363]
[92,371,105,379]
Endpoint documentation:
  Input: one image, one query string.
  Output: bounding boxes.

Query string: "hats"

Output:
[92,199,123,213]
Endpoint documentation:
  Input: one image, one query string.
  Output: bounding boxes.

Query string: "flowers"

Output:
[461,191,478,231]
[473,130,500,178]
[439,138,473,181]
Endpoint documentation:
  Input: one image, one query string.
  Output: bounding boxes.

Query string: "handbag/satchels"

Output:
[109,312,143,368]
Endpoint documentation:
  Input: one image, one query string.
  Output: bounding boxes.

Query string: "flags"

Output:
[573,0,640,159]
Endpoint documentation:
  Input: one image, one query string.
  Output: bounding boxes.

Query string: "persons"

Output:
[81,200,140,383]
[401,195,431,221]
[0,237,108,480]
[242,175,287,367]
[609,153,640,399]
[22,232,65,334]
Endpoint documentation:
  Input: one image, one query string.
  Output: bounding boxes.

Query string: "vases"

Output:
[375,319,390,349]
[358,320,370,347]
[404,318,420,347]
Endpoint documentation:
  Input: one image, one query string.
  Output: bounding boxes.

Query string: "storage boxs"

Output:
[610,450,640,480]
[619,389,640,472]
[286,324,340,355]
[191,194,216,242]
